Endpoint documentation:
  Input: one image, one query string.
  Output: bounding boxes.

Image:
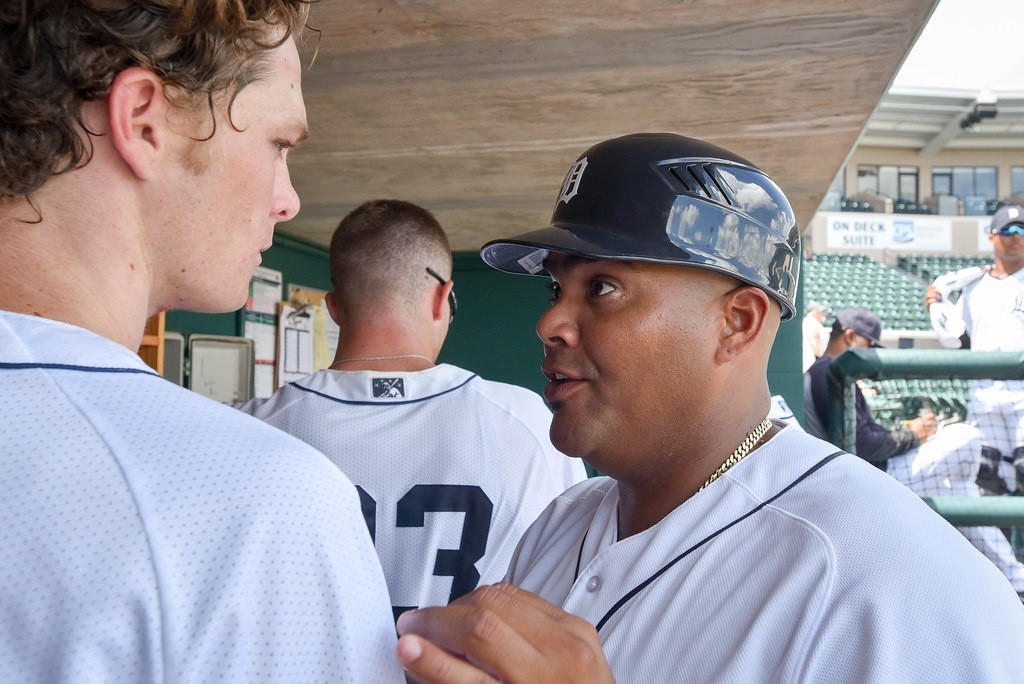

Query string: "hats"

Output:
[834,308,886,350]
[989,202,1024,237]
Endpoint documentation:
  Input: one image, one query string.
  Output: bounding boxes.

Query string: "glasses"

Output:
[426,266,457,323]
[990,223,1024,236]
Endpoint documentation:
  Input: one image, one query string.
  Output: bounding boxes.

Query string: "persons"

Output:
[0,0,406,684]
[395,133,1024,684]
[230,198,588,640]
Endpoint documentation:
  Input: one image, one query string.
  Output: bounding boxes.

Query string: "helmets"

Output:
[479,131,805,319]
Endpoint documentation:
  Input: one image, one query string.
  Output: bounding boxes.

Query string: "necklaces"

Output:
[331,354,435,366]
[698,418,772,494]
